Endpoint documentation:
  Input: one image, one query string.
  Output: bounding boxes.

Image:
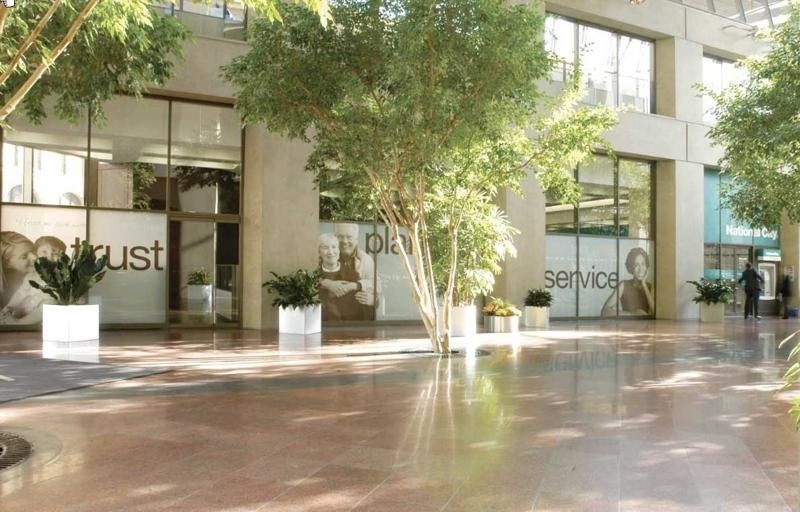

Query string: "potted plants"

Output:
[260,264,328,335]
[685,274,738,324]
[179,266,214,314]
[521,287,553,334]
[26,234,112,363]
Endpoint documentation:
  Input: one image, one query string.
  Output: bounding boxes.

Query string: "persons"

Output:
[600,248,656,319]
[777,265,795,321]
[10,185,83,209]
[1,232,86,325]
[318,222,381,322]
[736,261,764,321]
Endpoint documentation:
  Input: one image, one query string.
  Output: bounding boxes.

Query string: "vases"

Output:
[483,314,520,333]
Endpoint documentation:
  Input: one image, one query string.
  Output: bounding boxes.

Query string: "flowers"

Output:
[480,296,521,318]
